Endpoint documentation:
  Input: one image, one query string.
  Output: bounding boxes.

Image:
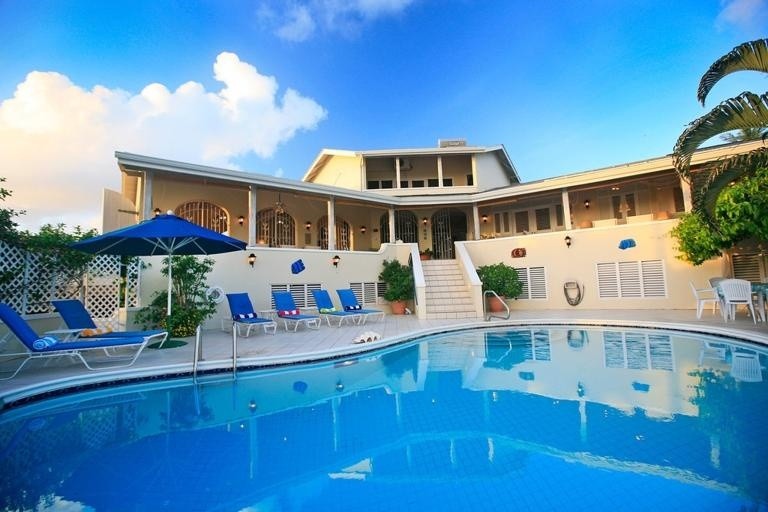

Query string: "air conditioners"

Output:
[394,158,411,171]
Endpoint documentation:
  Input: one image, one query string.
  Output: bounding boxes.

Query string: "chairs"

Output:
[336,288,385,324]
[719,279,756,324]
[312,289,362,328]
[0,303,148,381]
[221,293,277,337]
[52,299,169,358]
[272,292,321,332]
[689,282,724,319]
[708,277,737,317]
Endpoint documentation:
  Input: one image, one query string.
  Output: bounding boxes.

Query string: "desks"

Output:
[715,283,768,322]
[44,329,85,369]
[258,310,279,333]
[299,307,321,328]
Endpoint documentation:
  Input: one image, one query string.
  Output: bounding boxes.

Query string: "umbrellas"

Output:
[66,208,247,341]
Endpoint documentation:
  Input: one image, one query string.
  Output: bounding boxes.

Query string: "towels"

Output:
[33,336,58,349]
[80,326,113,337]
[234,304,363,319]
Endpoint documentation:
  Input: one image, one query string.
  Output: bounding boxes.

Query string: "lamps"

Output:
[481,214,488,222]
[153,208,367,268]
[584,199,590,208]
[422,217,428,225]
[565,236,571,248]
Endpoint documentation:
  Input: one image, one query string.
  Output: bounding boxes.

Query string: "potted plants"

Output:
[378,259,414,314]
[419,248,433,260]
[476,262,523,312]
